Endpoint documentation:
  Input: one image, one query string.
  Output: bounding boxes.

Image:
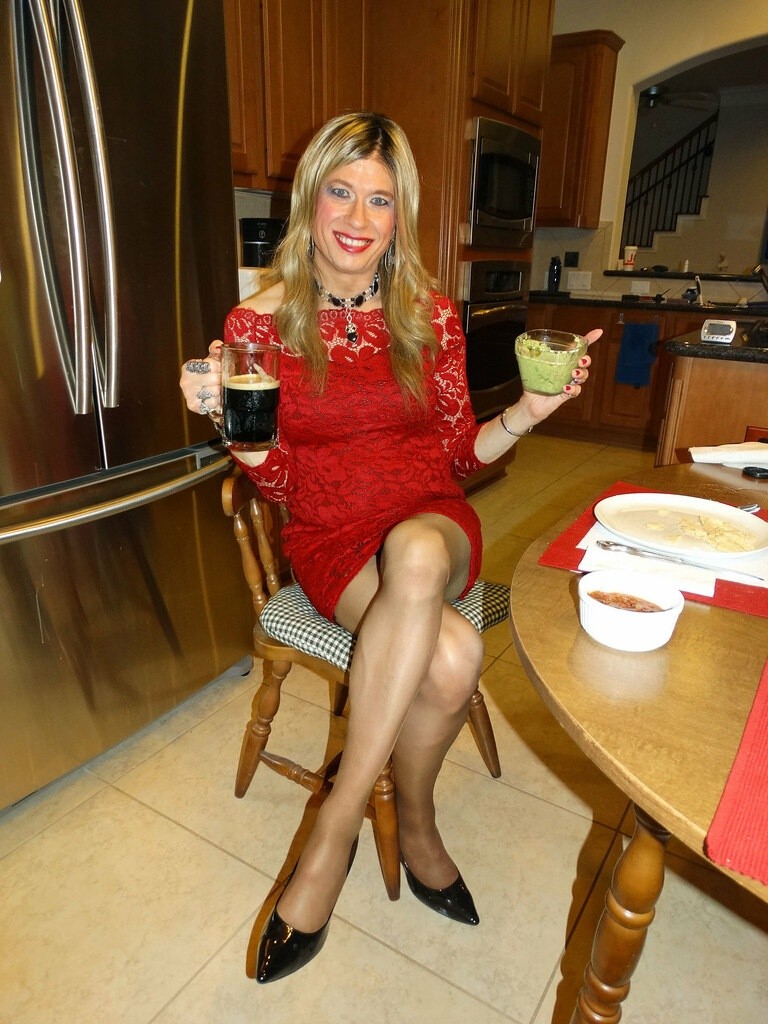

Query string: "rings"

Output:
[186,361,212,374]
[573,378,581,384]
[200,399,217,414]
[197,386,215,398]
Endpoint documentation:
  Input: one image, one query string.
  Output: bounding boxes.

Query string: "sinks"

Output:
[745,328,768,349]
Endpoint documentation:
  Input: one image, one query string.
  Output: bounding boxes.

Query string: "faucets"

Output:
[752,263,768,294]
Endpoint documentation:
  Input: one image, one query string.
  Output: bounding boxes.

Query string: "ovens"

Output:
[456,259,532,418]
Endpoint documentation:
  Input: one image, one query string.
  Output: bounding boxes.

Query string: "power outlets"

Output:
[566,271,591,290]
[631,281,650,295]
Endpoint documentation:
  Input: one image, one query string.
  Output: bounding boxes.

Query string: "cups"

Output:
[515,328,588,396]
[203,342,281,450]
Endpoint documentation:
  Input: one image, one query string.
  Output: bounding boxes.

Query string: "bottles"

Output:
[548,256,561,291]
[624,246,638,271]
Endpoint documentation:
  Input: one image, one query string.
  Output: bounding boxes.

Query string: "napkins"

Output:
[578,539,716,598]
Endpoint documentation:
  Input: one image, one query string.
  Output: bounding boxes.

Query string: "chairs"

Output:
[221,463,511,902]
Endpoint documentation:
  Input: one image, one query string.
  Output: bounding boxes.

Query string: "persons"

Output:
[179,111,604,984]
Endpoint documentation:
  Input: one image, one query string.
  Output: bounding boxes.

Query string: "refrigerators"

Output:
[0,0,253,815]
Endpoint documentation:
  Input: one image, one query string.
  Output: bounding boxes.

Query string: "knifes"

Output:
[596,540,764,583]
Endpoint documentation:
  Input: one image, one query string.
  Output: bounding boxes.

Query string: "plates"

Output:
[594,493,768,556]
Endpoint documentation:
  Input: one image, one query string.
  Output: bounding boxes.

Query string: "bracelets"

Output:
[501,408,532,437]
[213,422,231,447]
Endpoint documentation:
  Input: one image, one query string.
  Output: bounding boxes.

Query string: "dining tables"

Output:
[509,453,768,1024]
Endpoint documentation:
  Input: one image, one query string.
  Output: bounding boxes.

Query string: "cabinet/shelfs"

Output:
[525,302,768,451]
[222,0,627,230]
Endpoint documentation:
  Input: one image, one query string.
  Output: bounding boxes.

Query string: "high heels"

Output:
[398,851,481,927]
[255,832,360,984]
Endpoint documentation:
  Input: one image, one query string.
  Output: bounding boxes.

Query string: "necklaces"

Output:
[314,269,380,342]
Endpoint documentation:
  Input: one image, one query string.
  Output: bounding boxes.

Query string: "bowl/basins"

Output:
[577,569,684,653]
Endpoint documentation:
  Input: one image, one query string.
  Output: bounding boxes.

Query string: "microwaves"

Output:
[468,116,541,252]
